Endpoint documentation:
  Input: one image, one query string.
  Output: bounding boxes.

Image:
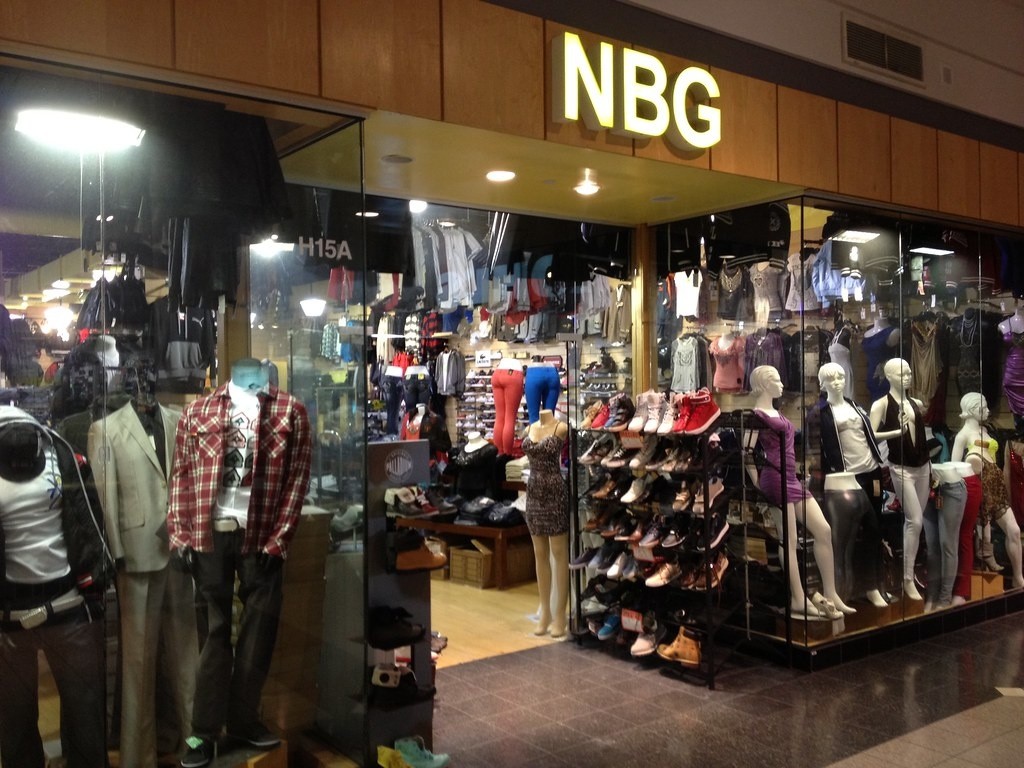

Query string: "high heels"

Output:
[976,540,1005,572]
[812,591,843,622]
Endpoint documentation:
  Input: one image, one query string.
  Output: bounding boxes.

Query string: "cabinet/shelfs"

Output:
[565,409,753,689]
[455,376,587,450]
[742,408,794,668]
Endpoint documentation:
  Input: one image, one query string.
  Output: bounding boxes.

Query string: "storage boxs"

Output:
[424,534,466,582]
[448,540,535,590]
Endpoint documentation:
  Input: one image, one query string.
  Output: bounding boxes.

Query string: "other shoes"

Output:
[426,488,457,515]
[568,386,739,669]
[384,487,424,520]
[406,486,440,517]
[394,734,450,768]
[181,730,210,767]
[371,664,437,711]
[514,394,530,440]
[368,604,426,650]
[456,369,496,447]
[385,528,447,573]
[464,355,474,361]
[577,357,632,394]
[227,722,281,751]
[376,744,412,767]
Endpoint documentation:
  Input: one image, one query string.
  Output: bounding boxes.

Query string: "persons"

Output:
[490,358,523,456]
[522,409,568,638]
[164,357,313,767]
[524,362,560,425]
[400,403,452,481]
[86,358,201,768]
[0,404,118,768]
[403,366,434,422]
[381,365,403,440]
[451,431,499,499]
[704,248,1024,614]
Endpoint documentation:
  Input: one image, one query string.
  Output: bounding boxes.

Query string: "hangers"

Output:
[394,280,993,354]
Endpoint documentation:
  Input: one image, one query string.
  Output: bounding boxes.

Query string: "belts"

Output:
[212,517,243,532]
[1,587,84,631]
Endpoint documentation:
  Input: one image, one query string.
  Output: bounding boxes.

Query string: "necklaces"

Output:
[406,419,421,434]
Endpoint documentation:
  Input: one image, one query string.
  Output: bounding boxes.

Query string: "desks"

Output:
[397,518,529,590]
